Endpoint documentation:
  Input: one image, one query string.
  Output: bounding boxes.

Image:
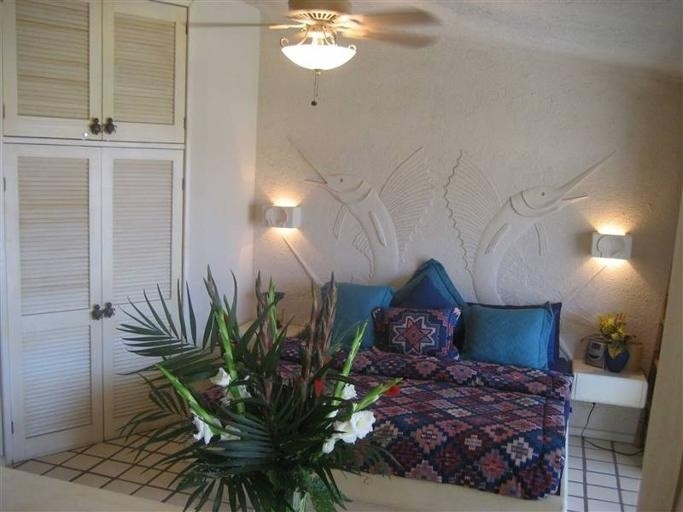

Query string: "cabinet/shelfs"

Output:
[0,144,187,466]
[2,3,187,150]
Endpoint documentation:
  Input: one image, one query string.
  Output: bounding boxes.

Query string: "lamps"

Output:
[280,0,357,107]
[592,233,632,260]
[265,206,302,227]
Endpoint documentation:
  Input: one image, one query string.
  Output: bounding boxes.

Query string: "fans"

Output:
[184,0,445,49]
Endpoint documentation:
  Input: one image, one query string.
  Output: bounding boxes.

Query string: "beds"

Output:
[265,344,574,512]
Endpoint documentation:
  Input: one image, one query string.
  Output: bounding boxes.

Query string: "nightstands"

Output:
[238,318,308,376]
[567,360,649,443]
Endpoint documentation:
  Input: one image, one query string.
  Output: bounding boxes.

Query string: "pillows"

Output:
[465,302,562,364]
[321,280,393,349]
[388,262,465,306]
[370,306,461,363]
[463,301,554,370]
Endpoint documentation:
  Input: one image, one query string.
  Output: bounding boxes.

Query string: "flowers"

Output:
[115,263,404,512]
[596,312,636,344]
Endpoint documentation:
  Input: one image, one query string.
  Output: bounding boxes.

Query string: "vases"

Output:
[605,345,630,373]
